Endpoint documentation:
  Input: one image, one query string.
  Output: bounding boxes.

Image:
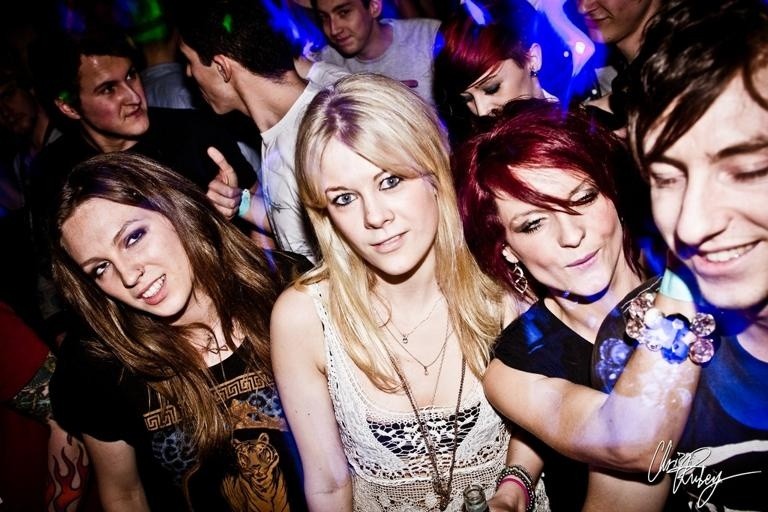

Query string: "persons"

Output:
[1,0,768,512]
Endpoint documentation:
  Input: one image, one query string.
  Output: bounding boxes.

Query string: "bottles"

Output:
[462,485,489,512]
